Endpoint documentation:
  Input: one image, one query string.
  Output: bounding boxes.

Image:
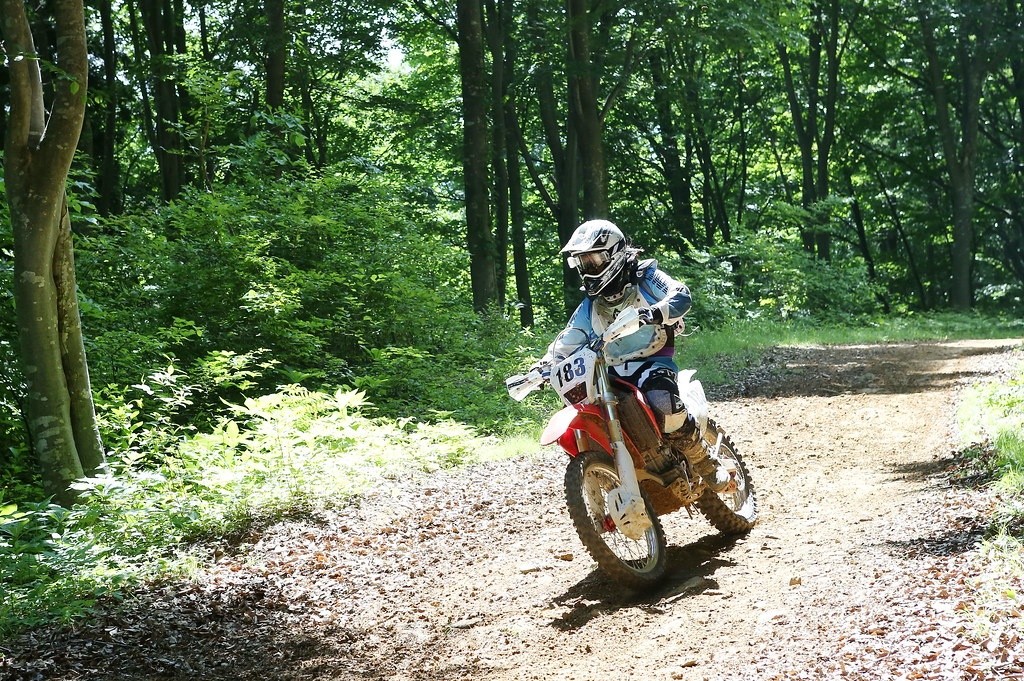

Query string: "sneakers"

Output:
[693,448,731,493]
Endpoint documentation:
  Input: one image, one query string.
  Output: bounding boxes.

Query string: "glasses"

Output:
[567,249,610,272]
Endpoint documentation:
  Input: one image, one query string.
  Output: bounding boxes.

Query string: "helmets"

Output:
[559,219,627,297]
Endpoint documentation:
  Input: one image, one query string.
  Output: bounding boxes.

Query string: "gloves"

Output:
[637,305,663,329]
[528,367,544,390]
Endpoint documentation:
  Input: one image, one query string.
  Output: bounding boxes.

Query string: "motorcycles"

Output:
[505,304,760,594]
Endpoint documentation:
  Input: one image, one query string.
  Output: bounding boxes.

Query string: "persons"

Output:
[530,219,730,492]
